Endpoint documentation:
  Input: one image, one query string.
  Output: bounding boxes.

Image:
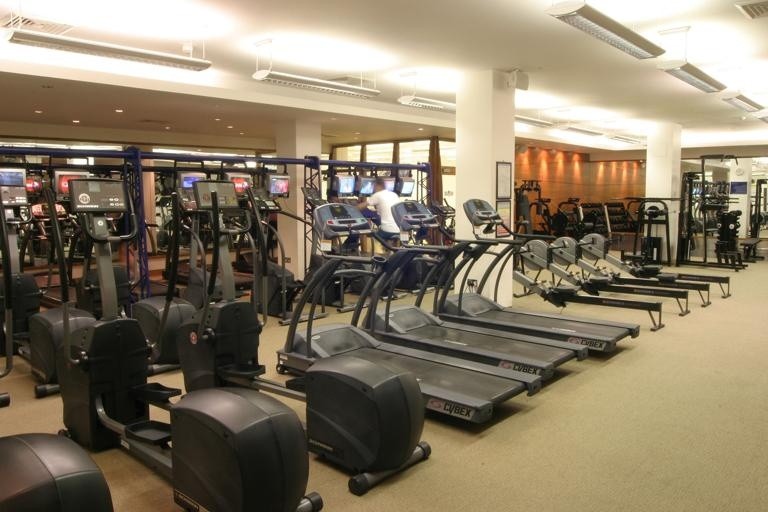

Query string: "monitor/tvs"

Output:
[272,177,290,195]
[230,176,251,195]
[0,169,27,189]
[55,172,89,200]
[332,175,415,198]
[730,182,747,194]
[180,175,206,190]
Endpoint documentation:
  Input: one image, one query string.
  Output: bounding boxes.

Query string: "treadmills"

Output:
[277,199,544,425]
[357,198,591,388]
[430,198,641,357]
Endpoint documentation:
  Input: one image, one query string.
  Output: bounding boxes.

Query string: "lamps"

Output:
[552,1,665,64]
[658,27,768,126]
[7,0,213,69]
[401,73,605,138]
[253,32,381,98]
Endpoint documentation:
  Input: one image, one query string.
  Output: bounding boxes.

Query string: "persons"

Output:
[356,178,403,255]
[30,190,67,239]
